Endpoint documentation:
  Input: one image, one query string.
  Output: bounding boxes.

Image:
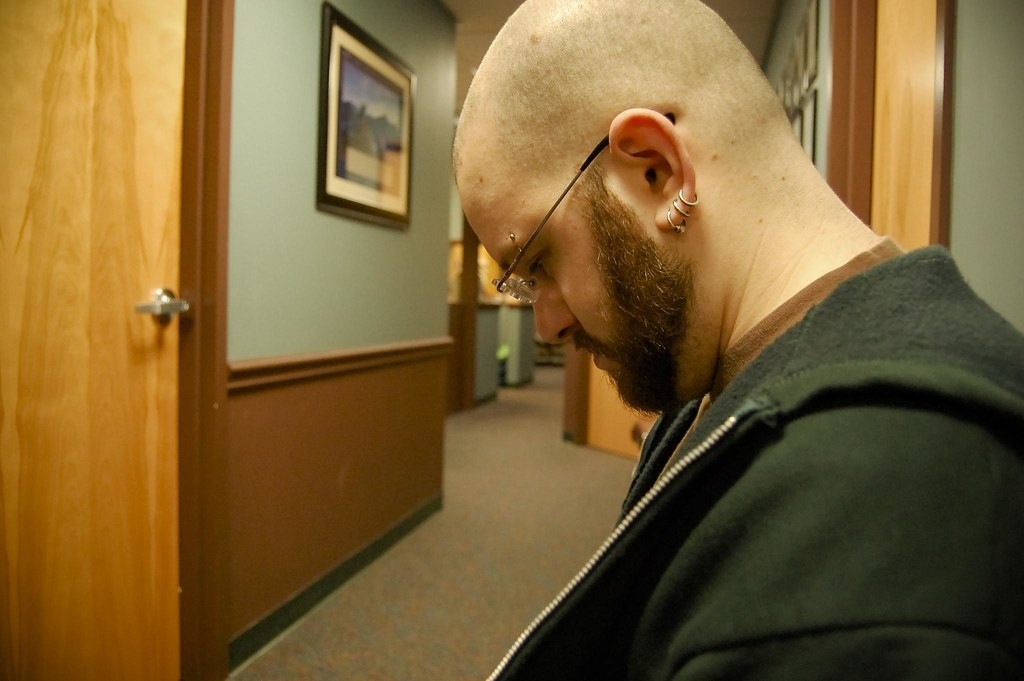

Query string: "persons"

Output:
[451,1,1024,681]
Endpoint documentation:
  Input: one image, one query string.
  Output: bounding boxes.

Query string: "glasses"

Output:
[491,112,676,304]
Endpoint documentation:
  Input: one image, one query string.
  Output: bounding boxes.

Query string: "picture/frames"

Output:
[770,0,817,165]
[315,0,418,229]
